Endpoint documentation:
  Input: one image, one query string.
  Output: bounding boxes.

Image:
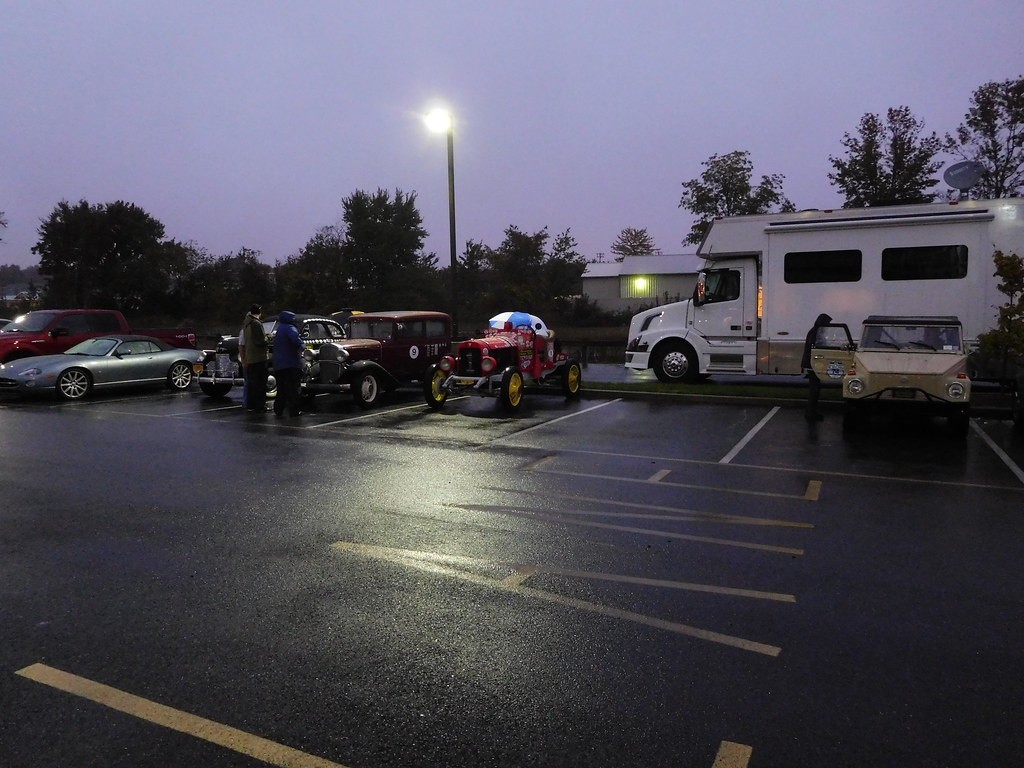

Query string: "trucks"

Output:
[625,197,1024,383]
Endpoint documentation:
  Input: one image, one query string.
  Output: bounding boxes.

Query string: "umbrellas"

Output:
[489,311,548,337]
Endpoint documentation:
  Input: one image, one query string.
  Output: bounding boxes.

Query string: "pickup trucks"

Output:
[0,309,196,365]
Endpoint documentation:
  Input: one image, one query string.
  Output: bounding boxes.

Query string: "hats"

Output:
[251,304,262,315]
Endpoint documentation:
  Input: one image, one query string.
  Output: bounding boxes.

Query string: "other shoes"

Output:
[805,412,825,421]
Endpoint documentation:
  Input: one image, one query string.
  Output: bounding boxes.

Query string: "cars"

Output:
[810,315,971,435]
[0,335,206,401]
[300,310,452,407]
[193,314,347,398]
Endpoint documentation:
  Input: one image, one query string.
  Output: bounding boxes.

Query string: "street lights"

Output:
[428,109,459,342]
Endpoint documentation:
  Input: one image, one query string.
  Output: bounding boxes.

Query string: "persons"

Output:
[799,313,833,422]
[271,311,306,418]
[237,304,268,420]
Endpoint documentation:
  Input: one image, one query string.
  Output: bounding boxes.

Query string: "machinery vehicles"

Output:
[424,321,581,411]
